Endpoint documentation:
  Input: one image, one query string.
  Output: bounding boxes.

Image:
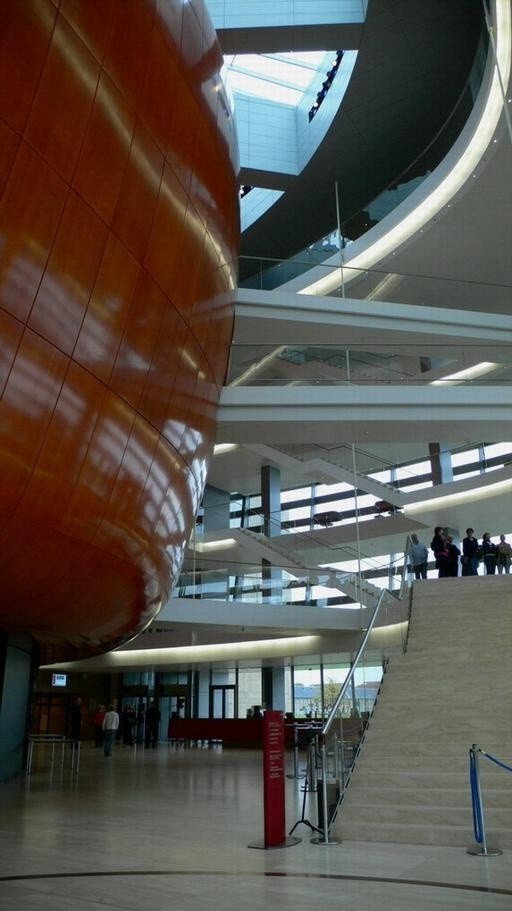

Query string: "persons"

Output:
[92,704,107,748]
[430,526,450,577]
[102,705,120,756]
[463,528,480,576]
[409,533,429,581]
[245,708,253,719]
[495,534,512,574]
[480,532,497,574]
[444,535,461,576]
[135,703,147,744]
[144,700,161,749]
[121,705,137,746]
[68,696,87,749]
